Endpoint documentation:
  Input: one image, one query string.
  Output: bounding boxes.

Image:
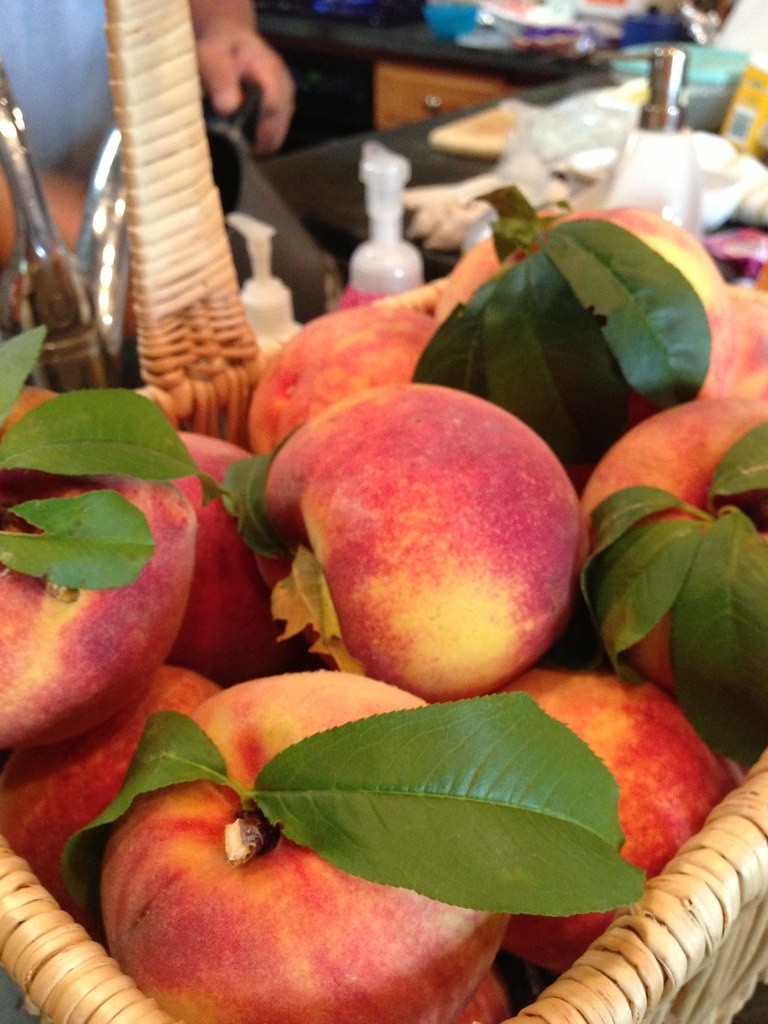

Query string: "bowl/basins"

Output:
[423,4,481,39]
[616,43,749,134]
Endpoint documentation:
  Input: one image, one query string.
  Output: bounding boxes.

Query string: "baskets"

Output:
[0,0,768,1024]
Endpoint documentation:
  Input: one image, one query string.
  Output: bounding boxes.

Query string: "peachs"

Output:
[0,202,768,1024]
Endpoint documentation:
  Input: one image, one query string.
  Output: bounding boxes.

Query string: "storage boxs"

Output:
[719,52,768,160]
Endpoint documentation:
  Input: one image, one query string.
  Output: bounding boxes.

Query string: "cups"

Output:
[203,124,335,317]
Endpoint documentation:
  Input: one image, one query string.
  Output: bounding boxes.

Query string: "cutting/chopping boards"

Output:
[429,107,516,159]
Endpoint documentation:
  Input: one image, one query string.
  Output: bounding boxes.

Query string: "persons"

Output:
[1,0,295,338]
